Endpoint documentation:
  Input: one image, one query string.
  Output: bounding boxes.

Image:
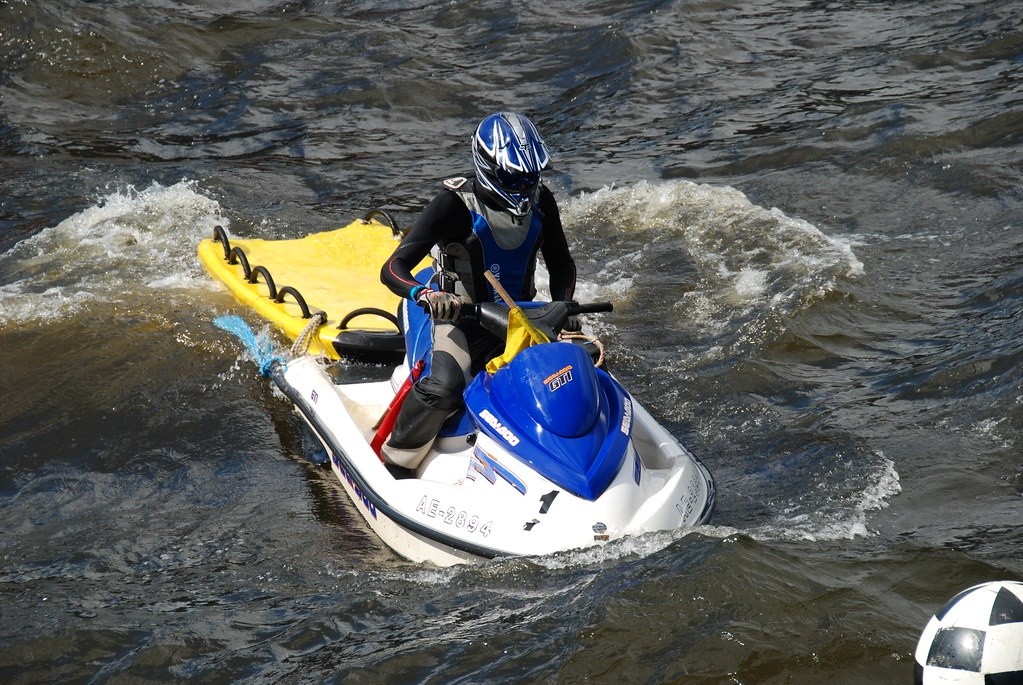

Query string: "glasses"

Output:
[500,169,539,190]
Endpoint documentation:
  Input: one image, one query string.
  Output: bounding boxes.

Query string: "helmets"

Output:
[472,113,551,221]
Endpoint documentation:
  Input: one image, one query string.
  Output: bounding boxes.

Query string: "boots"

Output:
[381,382,449,481]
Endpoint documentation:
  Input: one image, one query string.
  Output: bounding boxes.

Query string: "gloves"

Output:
[563,313,581,332]
[417,288,460,324]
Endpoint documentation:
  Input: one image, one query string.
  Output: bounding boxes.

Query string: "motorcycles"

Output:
[197,212,717,567]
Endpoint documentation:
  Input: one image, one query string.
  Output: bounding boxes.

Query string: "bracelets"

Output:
[410,285,427,302]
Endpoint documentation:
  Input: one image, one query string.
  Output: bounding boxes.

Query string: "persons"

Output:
[380,113,582,479]
